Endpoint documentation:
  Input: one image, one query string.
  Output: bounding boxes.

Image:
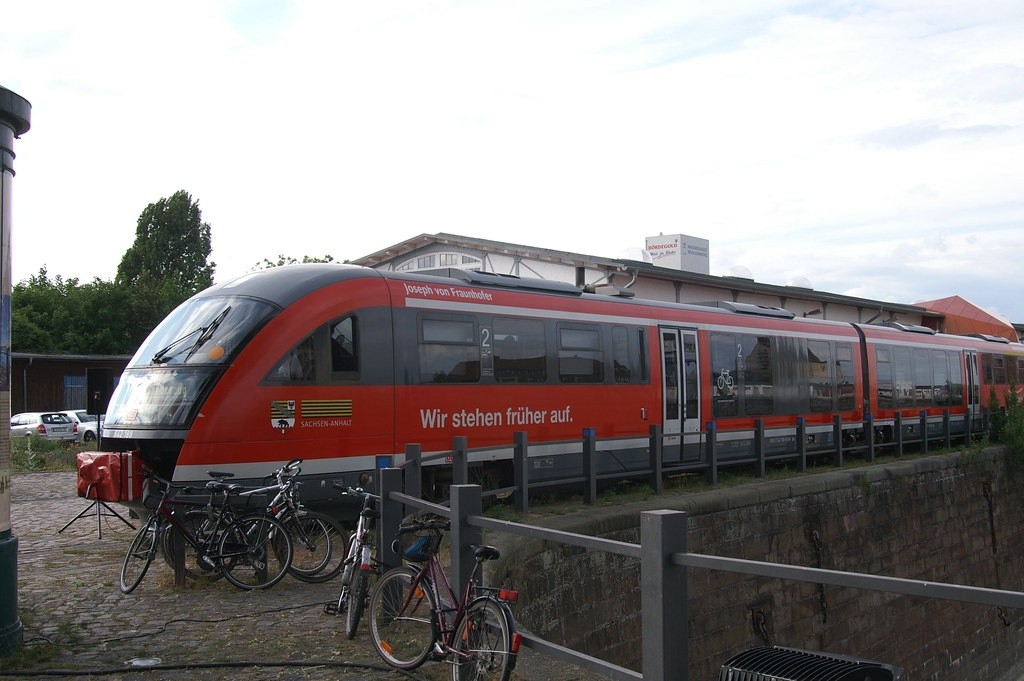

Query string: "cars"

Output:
[8,412,78,443]
[89,414,106,421]
[58,410,104,442]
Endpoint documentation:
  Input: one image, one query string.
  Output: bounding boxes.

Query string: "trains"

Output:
[75,261,1024,528]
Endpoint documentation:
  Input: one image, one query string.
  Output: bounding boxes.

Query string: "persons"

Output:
[273,352,304,381]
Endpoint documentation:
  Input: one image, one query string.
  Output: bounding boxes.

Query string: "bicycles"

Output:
[120,456,351,594]
[324,482,380,639]
[368,519,523,681]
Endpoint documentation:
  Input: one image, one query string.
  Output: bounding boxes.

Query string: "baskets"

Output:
[143,479,174,511]
[399,528,444,563]
[267,489,297,510]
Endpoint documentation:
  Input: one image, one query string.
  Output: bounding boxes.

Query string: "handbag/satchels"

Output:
[404,537,430,561]
